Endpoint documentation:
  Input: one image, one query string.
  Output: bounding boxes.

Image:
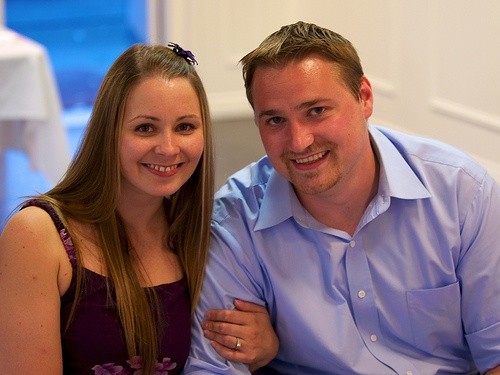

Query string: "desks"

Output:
[0,26,73,187]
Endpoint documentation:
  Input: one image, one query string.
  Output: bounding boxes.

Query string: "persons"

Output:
[184,21,500,375]
[0,40,281,375]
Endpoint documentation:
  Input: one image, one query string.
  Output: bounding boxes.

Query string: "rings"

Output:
[236,338,241,351]
